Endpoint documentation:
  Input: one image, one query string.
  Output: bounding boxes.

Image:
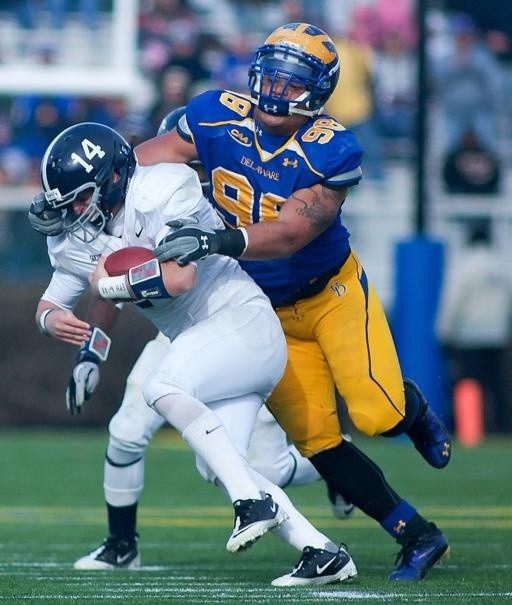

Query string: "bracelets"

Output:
[40,308,52,340]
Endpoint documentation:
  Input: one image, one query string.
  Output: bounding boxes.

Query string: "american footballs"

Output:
[105,247,154,276]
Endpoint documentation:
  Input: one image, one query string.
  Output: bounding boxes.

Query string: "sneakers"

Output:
[328,481,355,519]
[227,494,288,553]
[74,534,141,571]
[390,527,449,580]
[403,379,452,468]
[271,542,358,585]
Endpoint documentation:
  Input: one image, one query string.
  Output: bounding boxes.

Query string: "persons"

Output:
[0,0,512,247]
[156,106,357,520]
[33,122,360,590]
[25,20,454,583]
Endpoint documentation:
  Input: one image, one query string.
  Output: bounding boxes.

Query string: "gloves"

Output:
[65,328,111,416]
[29,193,63,236]
[153,218,248,267]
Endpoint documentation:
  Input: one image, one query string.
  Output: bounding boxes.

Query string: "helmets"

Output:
[39,123,136,224]
[249,22,339,116]
